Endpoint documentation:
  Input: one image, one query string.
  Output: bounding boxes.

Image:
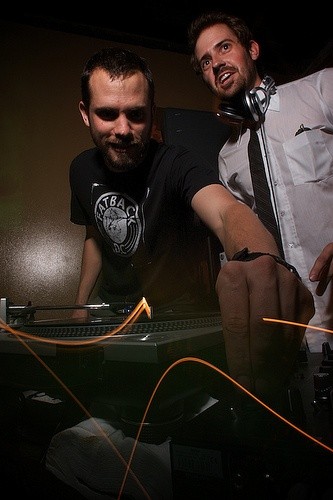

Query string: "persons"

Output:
[191,21,332,369]
[67,56,316,396]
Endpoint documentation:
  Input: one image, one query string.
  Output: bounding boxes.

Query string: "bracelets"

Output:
[230,246,301,280]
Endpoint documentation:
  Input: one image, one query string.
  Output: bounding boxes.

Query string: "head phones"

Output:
[215,73,277,131]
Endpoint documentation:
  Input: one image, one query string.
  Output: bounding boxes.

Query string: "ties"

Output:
[248,126,285,260]
